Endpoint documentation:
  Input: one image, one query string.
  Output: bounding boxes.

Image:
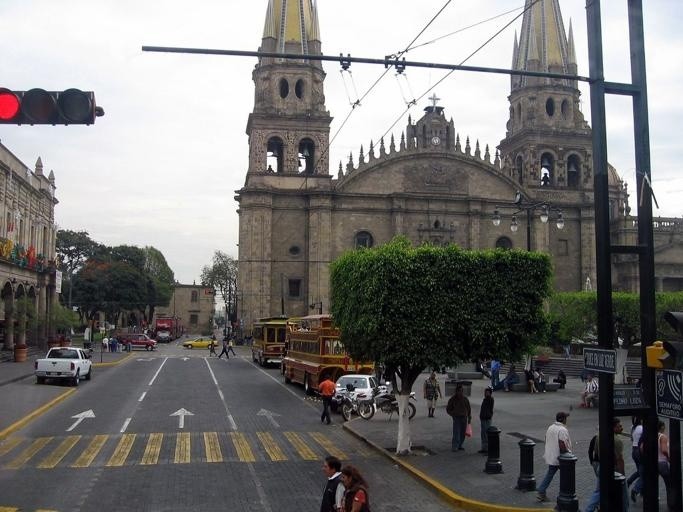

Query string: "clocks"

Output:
[430,136,440,145]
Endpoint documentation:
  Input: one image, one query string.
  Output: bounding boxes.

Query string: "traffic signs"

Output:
[613,383,650,410]
[582,348,616,374]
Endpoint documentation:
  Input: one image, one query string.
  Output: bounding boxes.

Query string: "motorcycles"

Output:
[337,386,364,422]
[356,384,417,421]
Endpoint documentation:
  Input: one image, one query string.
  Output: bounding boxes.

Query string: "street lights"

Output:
[309,301,322,315]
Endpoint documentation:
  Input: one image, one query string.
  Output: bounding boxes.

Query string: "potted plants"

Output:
[2,292,45,362]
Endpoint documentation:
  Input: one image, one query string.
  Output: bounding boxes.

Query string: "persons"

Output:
[268,165,272,172]
[103,335,132,353]
[541,173,550,185]
[478,388,494,455]
[320,456,345,512]
[582,414,670,512]
[423,371,442,417]
[480,358,566,394]
[537,412,572,502]
[318,374,336,424]
[208,334,236,359]
[578,373,599,409]
[446,386,471,452]
[340,463,371,512]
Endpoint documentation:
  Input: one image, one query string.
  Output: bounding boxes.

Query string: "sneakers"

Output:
[536,494,551,502]
[452,447,489,454]
[630,488,638,503]
[321,419,331,424]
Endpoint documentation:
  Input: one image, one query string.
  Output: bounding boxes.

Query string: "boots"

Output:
[428,408,435,417]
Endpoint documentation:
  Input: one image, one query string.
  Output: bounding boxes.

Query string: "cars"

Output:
[153,331,172,342]
[131,325,152,334]
[181,336,219,350]
[327,372,378,412]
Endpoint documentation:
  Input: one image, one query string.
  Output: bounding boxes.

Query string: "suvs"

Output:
[118,332,158,352]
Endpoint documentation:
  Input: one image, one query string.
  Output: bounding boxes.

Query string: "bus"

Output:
[279,313,375,395]
[249,315,290,367]
[153,315,184,339]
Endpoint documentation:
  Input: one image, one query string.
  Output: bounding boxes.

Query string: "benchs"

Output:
[590,391,599,407]
[508,372,562,394]
[447,368,484,379]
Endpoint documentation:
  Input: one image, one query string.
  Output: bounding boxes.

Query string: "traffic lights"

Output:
[0,84,99,128]
[644,340,671,369]
[657,310,682,371]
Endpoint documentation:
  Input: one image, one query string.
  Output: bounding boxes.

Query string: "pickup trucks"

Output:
[33,345,93,388]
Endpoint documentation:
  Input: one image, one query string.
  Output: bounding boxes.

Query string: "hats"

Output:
[556,411,570,422]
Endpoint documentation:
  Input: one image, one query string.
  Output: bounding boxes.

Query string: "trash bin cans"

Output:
[445,380,472,397]
[84,343,91,349]
